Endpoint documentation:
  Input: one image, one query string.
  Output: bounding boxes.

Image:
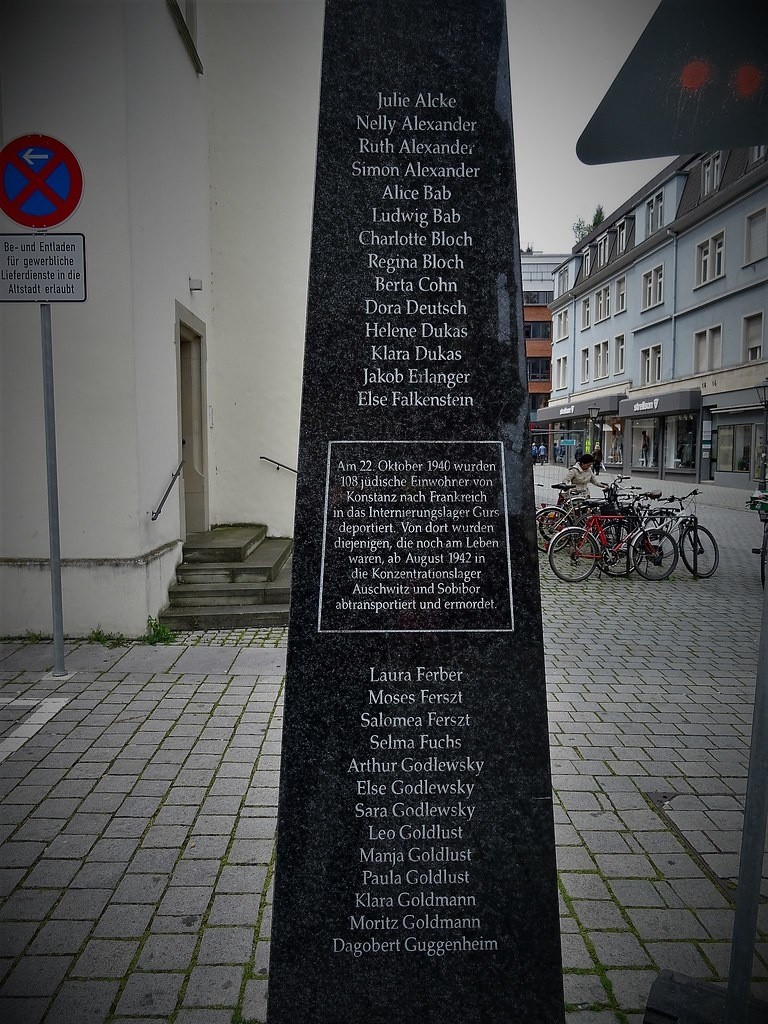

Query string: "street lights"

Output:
[587,402,601,474]
[754,377,768,445]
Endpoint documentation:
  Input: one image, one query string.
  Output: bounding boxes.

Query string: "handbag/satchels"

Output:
[557,490,567,505]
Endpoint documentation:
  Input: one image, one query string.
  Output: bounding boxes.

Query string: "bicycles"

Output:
[535,475,720,582]
[745,494,767,591]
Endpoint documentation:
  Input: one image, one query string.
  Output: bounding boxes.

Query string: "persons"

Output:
[641,430,649,467]
[616,430,624,465]
[553,443,559,464]
[562,453,608,507]
[539,442,546,465]
[532,443,538,466]
[574,443,583,464]
[610,431,618,463]
[592,445,602,476]
